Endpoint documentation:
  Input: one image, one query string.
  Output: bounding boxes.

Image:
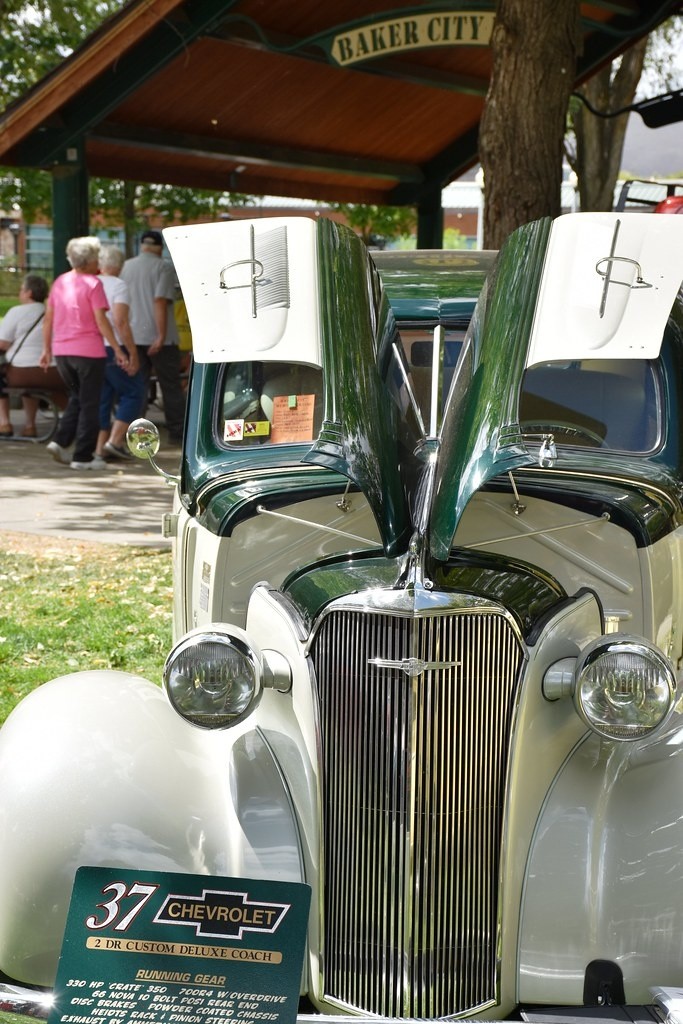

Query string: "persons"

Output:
[38,236,129,469]
[0,275,68,437]
[117,230,188,445]
[95,247,144,463]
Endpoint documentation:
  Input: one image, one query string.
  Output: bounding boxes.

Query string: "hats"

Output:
[141,231,163,246]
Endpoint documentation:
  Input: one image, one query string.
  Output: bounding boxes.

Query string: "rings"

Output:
[126,361,129,364]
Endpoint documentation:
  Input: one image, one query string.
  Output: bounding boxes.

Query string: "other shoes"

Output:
[70,459,105,470]
[94,454,120,463]
[169,434,182,444]
[0,424,13,436]
[46,441,70,464]
[17,427,37,437]
[103,441,134,460]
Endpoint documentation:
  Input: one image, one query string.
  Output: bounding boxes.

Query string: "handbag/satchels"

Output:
[0,353,10,383]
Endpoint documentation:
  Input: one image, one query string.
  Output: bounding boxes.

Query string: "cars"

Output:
[0,211,683,1024]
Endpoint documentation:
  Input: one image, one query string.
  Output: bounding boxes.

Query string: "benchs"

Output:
[0,372,188,444]
